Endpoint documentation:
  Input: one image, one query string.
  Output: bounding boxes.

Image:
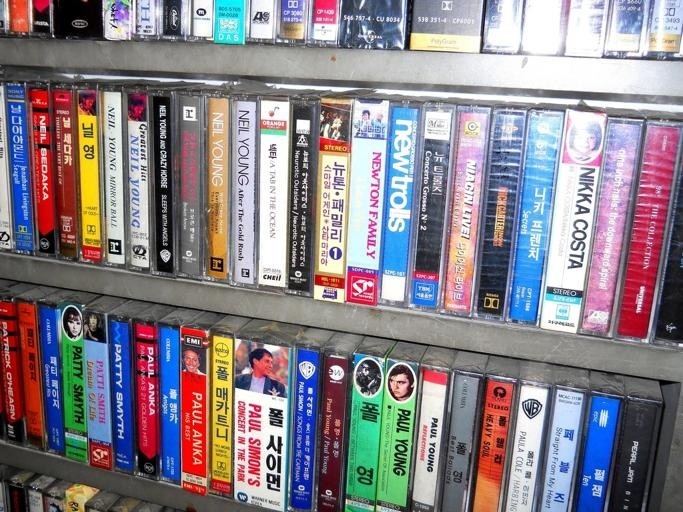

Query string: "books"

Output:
[1,81,682,344]
[1,1,683,61]
[0,462,182,512]
[1,278,662,511]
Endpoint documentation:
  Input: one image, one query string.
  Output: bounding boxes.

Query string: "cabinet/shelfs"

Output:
[1,28,683,512]
